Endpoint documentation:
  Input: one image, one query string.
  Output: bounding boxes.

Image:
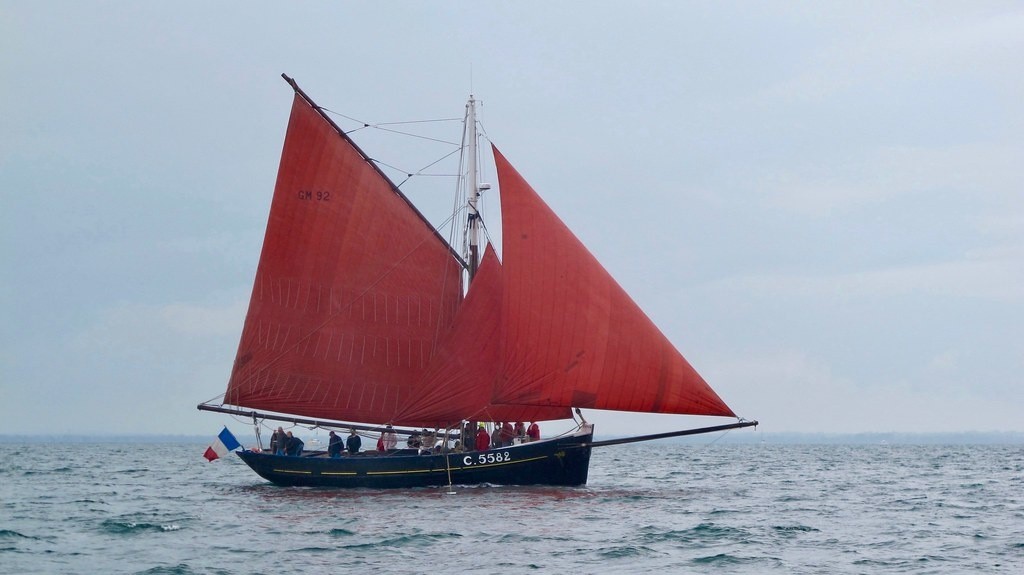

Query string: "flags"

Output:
[203,427,242,463]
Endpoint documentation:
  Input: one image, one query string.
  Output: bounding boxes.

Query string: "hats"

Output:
[478,424,484,428]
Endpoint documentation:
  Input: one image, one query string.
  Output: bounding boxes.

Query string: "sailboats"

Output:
[196,72,759,489]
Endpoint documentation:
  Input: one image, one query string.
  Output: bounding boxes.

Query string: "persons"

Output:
[270,421,541,456]
